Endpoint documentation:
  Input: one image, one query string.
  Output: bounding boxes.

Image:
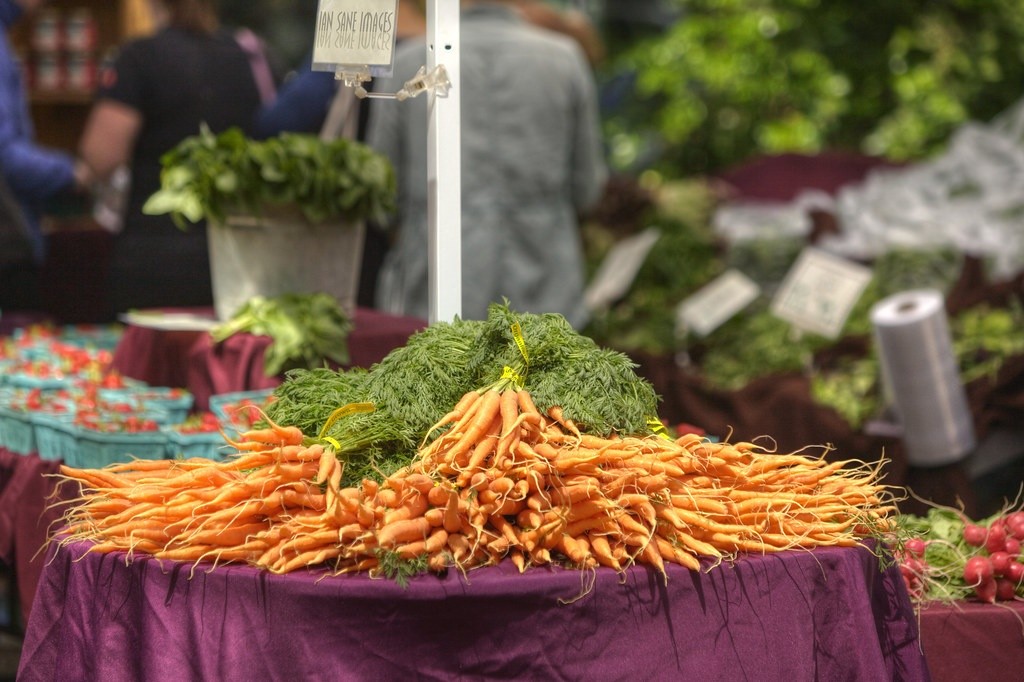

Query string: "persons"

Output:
[0,1,93,330]
[237,29,339,145]
[362,0,609,329]
[193,0,278,111]
[72,0,262,324]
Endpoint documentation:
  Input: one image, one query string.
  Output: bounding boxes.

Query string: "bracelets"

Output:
[246,50,266,60]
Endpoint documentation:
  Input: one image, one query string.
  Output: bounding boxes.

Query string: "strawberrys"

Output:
[0,322,278,434]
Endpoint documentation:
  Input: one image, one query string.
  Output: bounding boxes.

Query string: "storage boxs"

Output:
[0,325,279,471]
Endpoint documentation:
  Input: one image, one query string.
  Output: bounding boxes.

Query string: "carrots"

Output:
[39,388,908,585]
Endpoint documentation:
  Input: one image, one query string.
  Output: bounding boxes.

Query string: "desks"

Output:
[15,531,931,681]
[912,599,1024,681]
[109,306,427,421]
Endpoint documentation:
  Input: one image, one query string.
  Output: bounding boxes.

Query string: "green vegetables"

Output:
[571,1,1024,431]
[141,121,401,236]
[208,293,354,376]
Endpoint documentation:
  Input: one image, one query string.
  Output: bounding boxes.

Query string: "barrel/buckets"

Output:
[206,213,363,320]
